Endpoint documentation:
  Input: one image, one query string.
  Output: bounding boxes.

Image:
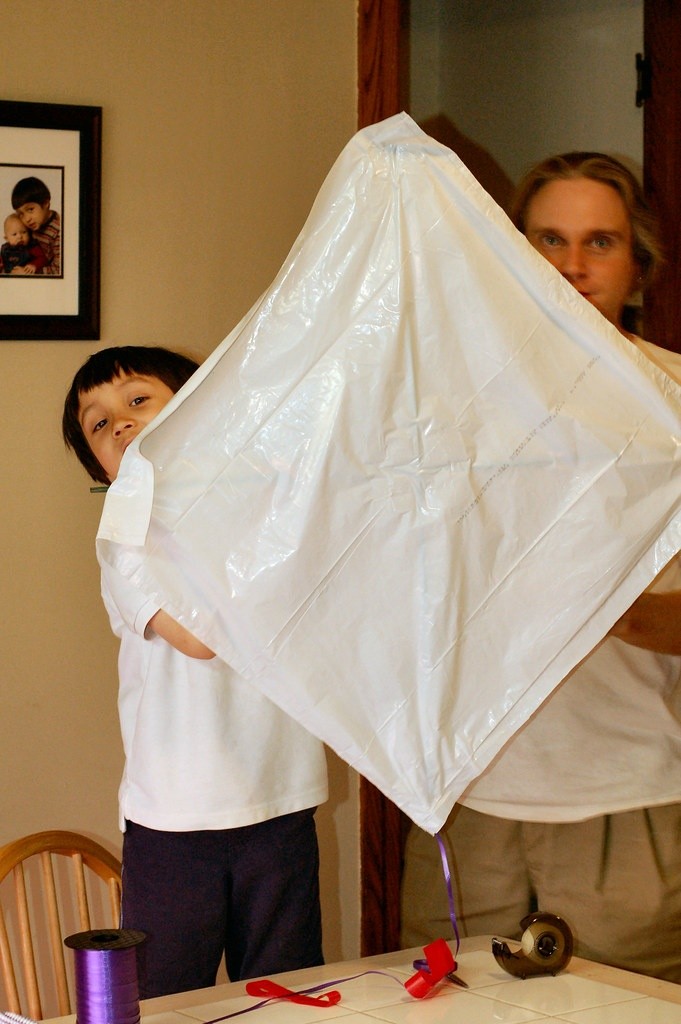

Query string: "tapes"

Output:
[520,921,565,967]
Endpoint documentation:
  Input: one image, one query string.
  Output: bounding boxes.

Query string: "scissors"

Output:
[413,959,469,990]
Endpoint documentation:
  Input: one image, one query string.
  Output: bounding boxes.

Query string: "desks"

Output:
[29,936,681,1024]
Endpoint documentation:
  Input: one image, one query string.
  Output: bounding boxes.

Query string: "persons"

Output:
[0,177,61,276]
[394,150,681,988]
[62,345,329,1000]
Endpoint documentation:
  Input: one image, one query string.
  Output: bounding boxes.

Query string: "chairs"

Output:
[0,828,122,1022]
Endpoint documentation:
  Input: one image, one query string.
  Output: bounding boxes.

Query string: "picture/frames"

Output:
[0,98,101,341]
